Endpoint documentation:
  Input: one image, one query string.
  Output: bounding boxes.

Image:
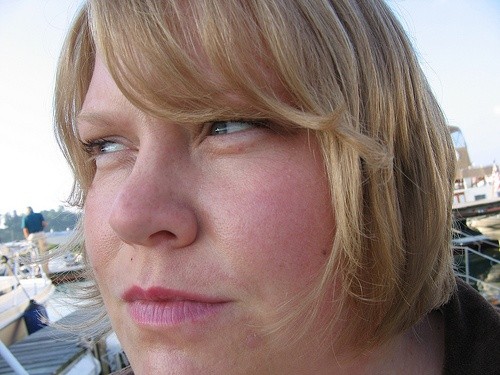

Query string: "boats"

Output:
[445,125,500,217]
[0,242,55,346]
[0,243,88,282]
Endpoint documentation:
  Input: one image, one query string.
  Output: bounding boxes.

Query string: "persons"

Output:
[20,206,50,275]
[50,1,500,375]
